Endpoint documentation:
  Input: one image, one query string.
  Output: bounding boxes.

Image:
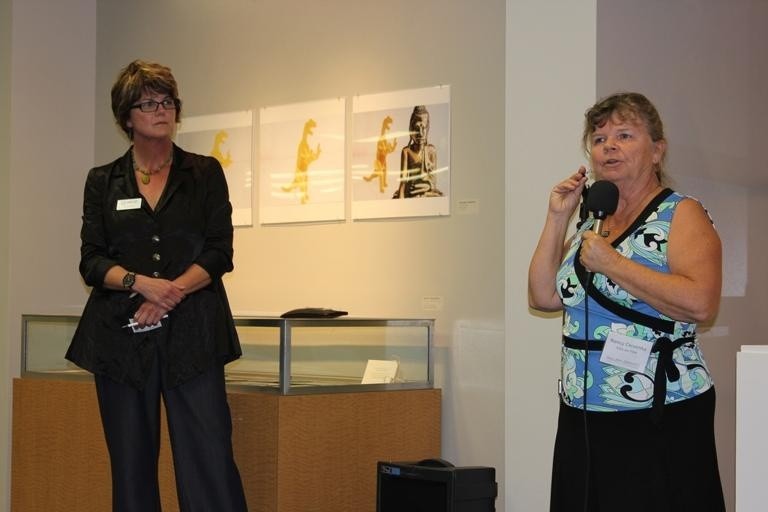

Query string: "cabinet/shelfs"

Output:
[10,314,443,512]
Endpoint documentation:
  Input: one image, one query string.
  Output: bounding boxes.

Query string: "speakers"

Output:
[376,458,498,512]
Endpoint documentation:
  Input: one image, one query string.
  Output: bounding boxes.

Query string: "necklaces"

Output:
[128,146,175,185]
[603,183,662,237]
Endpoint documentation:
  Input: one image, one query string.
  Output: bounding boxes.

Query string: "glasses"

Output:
[130,98,180,113]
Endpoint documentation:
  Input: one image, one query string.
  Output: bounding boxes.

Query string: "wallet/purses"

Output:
[280,308,348,319]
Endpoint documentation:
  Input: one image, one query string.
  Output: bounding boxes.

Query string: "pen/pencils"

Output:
[121,314,168,328]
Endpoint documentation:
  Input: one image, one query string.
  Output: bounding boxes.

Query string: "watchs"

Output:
[122,270,137,291]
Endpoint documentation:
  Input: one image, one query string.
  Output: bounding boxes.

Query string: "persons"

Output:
[392,105,444,198]
[521,90,725,511]
[62,55,251,510]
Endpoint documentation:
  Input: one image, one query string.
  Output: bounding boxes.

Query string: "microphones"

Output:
[585,180,619,289]
